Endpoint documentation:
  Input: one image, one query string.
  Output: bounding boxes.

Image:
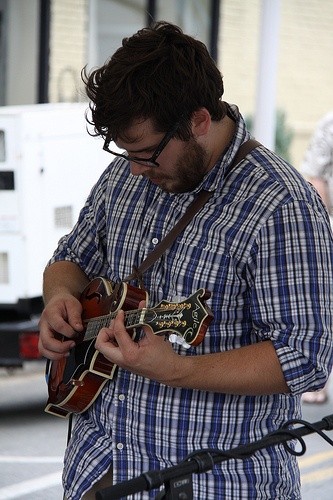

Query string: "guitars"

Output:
[44,274,214,422]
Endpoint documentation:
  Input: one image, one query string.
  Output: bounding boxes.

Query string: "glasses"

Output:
[102,127,177,167]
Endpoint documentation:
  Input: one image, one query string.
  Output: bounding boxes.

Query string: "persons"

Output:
[33,22,332,499]
